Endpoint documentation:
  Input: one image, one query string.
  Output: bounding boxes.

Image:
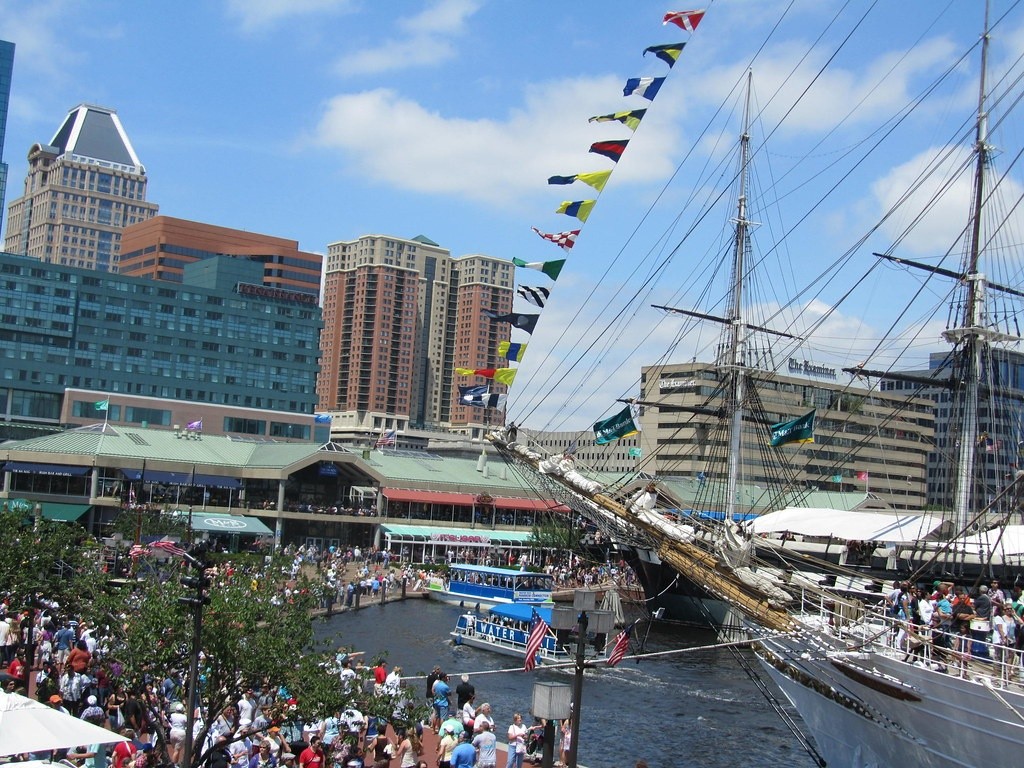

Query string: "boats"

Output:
[425,566,554,610]
[611,524,1016,630]
[450,598,616,666]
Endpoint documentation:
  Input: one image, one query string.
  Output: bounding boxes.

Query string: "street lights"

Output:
[178,554,211,768]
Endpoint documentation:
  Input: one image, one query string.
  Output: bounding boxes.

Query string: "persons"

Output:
[0,490,1024,768]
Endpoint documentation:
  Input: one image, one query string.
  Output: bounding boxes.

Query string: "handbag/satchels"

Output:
[389,747,396,760]
[290,737,309,764]
[516,743,526,755]
[52,642,59,652]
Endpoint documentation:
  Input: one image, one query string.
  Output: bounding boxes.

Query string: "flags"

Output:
[314,414,332,423]
[986,437,1001,455]
[833,475,842,483]
[767,408,817,447]
[95,399,108,410]
[524,607,548,674]
[629,448,642,457]
[695,472,706,481]
[377,430,395,445]
[857,471,868,480]
[606,624,636,668]
[593,405,641,445]
[186,420,203,430]
[456,9,706,408]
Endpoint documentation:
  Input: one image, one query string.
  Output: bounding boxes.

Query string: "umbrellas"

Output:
[0,692,132,755]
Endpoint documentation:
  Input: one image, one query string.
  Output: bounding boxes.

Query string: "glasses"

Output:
[260,747,266,749]
[437,668,440,670]
[242,732,248,734]
[992,582,999,584]
[911,588,915,589]
[1006,608,1012,610]
[9,683,15,685]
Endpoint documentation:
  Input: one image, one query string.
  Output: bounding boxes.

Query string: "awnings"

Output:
[0,498,93,523]
[160,510,274,537]
[378,524,558,544]
[120,468,244,490]
[1,463,91,476]
[382,488,571,513]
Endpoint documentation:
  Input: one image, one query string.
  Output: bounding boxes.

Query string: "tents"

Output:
[741,506,975,543]
[927,524,1024,555]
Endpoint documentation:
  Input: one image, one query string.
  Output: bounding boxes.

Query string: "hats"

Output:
[87,695,97,705]
[445,724,454,733]
[281,752,296,762]
[448,711,456,717]
[459,731,469,738]
[143,743,155,752]
[237,718,251,734]
[269,726,280,732]
[176,704,184,712]
[49,695,62,703]
[570,702,574,712]
[979,585,989,592]
[460,674,469,683]
[171,668,178,673]
[341,659,353,667]
[379,658,389,664]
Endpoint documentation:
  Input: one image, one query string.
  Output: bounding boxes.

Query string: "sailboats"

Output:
[487,0,1024,768]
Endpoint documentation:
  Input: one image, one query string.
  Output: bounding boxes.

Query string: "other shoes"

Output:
[432,731,438,735]
